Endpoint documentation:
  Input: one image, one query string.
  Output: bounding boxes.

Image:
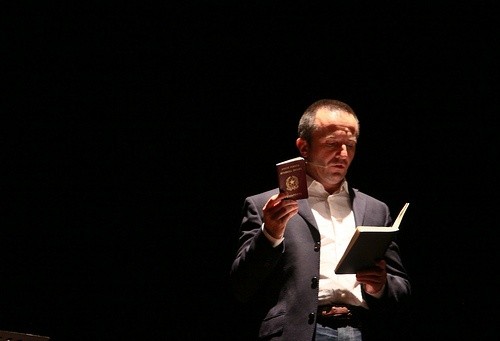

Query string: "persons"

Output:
[227,99,411,341]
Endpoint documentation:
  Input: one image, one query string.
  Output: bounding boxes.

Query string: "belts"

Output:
[316,304,365,321]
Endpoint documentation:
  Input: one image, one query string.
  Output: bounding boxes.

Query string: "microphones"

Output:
[306,162,328,168]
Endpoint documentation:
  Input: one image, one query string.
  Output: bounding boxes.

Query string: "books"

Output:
[275,157,309,201]
[332,201,409,274]
